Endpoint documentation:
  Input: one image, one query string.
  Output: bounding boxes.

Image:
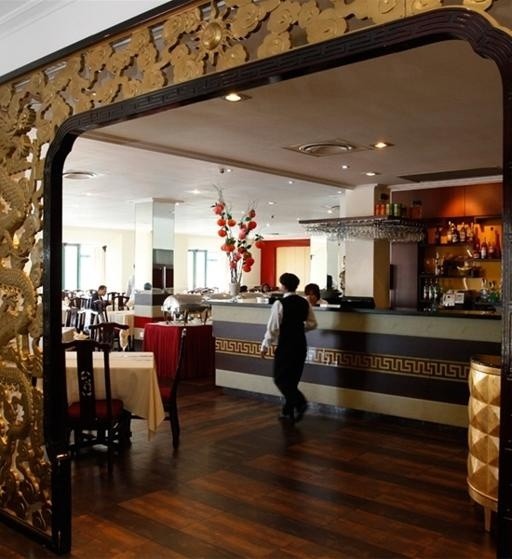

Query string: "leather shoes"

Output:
[296,404,307,422]
[279,414,294,419]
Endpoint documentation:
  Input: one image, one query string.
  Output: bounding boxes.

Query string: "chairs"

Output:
[32,284,217,495]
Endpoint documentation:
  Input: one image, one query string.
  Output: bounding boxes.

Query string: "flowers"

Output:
[207,179,266,281]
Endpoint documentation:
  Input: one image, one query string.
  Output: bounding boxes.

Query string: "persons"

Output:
[260,272,319,422]
[304,283,329,306]
[261,283,274,294]
[85,284,113,323]
[239,285,249,294]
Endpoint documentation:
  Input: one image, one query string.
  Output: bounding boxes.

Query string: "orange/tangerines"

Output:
[238,233,245,239]
[243,265,251,272]
[238,247,245,254]
[214,206,222,214]
[244,252,250,260]
[247,258,254,266]
[222,244,234,252]
[218,230,226,237]
[240,225,246,231]
[248,221,256,230]
[217,219,226,226]
[218,204,224,210]
[229,219,236,227]
[230,262,237,269]
[250,212,256,217]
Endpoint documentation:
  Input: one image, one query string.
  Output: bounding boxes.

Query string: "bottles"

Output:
[473,227,501,259]
[481,278,503,305]
[422,277,455,313]
[434,220,474,244]
[435,250,446,275]
[370,200,424,220]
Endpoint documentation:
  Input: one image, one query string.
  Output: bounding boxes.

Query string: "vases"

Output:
[228,283,240,296]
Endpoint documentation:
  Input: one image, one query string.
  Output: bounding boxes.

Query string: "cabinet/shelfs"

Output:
[392,175,503,218]
[422,237,502,279]
